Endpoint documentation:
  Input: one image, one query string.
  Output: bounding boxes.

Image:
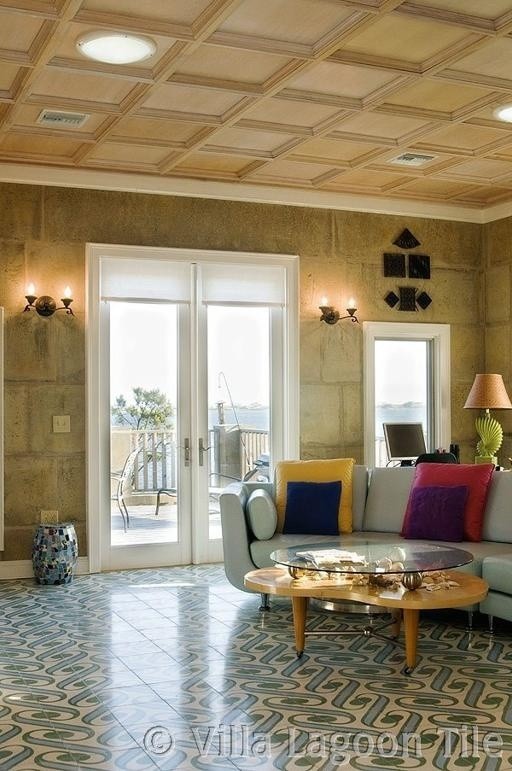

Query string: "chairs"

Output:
[111,446,141,535]
[155,467,258,517]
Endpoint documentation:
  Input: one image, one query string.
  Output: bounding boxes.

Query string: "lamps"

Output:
[319,297,359,325]
[24,281,74,317]
[463,373,512,420]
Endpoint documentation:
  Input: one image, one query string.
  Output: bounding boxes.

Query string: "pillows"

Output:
[400,460,496,544]
[246,457,356,540]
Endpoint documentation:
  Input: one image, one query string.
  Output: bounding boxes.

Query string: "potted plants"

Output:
[475,417,503,467]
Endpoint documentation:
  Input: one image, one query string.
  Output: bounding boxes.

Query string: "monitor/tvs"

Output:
[383,422,426,461]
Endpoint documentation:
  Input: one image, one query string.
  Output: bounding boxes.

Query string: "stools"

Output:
[31,524,80,585]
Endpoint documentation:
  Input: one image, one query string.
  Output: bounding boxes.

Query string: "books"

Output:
[293,547,369,566]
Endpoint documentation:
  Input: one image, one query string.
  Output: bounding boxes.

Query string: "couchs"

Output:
[218,463,512,635]
